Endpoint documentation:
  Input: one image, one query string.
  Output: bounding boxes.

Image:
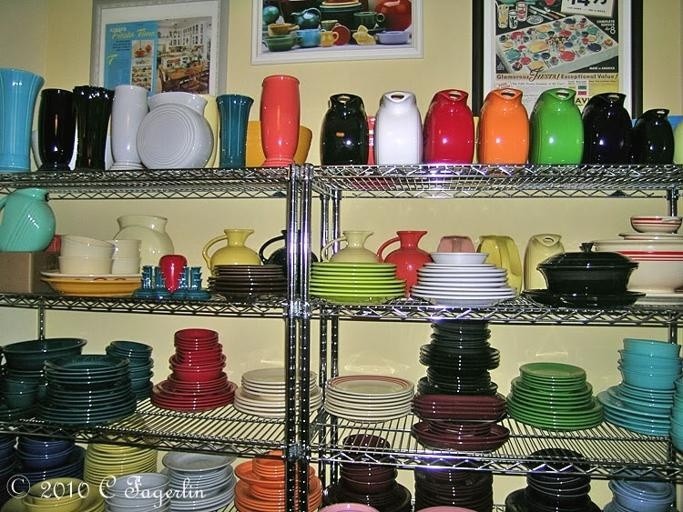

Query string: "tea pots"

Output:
[320,88,673,164]
[291,7,320,29]
[0,188,55,252]
[203,229,564,291]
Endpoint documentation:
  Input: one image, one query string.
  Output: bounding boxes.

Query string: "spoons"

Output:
[544,7,566,17]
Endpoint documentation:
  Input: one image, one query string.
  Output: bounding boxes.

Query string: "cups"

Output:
[321,20,338,30]
[497,2,527,29]
[353,11,385,29]
[265,23,339,52]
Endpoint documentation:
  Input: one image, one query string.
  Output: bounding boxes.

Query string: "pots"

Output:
[536,242,639,291]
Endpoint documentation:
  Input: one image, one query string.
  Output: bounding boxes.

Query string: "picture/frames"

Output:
[474,0,643,115]
[90,2,229,94]
[253,2,423,65]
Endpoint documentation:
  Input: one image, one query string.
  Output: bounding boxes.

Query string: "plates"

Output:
[35,321,682,451]
[320,0,361,8]
[527,15,544,25]
[207,263,516,309]
[499,17,614,72]
[38,269,142,296]
[520,289,646,310]
[1,431,494,512]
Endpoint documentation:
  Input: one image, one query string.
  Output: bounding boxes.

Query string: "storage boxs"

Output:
[0,251,59,293]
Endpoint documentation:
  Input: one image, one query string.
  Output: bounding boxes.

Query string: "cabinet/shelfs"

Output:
[1,170,288,461]
[308,165,681,481]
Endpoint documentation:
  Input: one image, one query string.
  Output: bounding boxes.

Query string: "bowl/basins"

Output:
[59,236,142,275]
[376,31,410,44]
[1,338,87,421]
[592,215,682,293]
[505,448,677,512]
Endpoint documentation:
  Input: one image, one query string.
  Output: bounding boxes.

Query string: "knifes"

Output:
[529,5,559,20]
